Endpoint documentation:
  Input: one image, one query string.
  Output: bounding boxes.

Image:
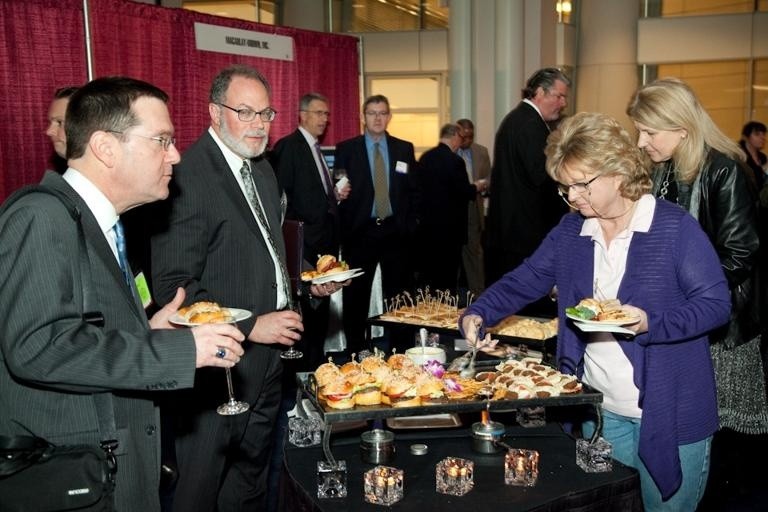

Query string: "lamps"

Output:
[553,0,577,72]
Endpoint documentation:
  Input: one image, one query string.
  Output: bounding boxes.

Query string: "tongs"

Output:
[447,325,493,380]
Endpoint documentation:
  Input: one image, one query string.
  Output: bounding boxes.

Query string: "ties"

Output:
[314,143,336,213]
[112,220,134,286]
[240,161,291,309]
[373,142,394,219]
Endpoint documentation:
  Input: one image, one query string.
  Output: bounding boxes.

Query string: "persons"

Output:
[270,91,351,366]
[334,94,416,354]
[406,121,491,307]
[458,112,731,512]
[456,118,491,254]
[36,85,81,184]
[738,121,767,175]
[0,71,245,511]
[146,64,351,511]
[625,73,768,435]
[479,67,571,318]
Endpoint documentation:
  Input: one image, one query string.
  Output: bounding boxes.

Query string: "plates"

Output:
[368,301,559,348]
[166,307,253,328]
[565,306,641,327]
[309,267,364,284]
[304,268,361,282]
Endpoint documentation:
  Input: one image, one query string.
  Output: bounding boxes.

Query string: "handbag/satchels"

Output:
[1,431,118,512]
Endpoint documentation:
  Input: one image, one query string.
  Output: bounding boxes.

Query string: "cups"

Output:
[278,300,304,360]
[207,315,251,417]
[333,168,346,184]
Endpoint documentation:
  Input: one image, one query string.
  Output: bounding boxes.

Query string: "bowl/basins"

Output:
[404,347,446,366]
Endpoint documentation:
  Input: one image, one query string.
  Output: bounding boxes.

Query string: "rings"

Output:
[216,347,228,360]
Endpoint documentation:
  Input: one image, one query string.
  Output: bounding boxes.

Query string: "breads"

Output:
[479,356,581,396]
[491,315,562,340]
[180,300,223,324]
[382,299,470,329]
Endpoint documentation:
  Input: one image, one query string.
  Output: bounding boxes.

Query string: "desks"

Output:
[283,424,643,512]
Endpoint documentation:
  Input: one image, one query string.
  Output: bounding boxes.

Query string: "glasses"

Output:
[304,110,329,115]
[364,111,388,117]
[106,126,182,150]
[552,173,600,194]
[215,102,276,123]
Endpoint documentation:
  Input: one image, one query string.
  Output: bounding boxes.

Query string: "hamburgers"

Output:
[317,255,343,275]
[419,379,447,406]
[387,377,419,407]
[356,370,382,406]
[314,354,428,377]
[322,373,355,410]
